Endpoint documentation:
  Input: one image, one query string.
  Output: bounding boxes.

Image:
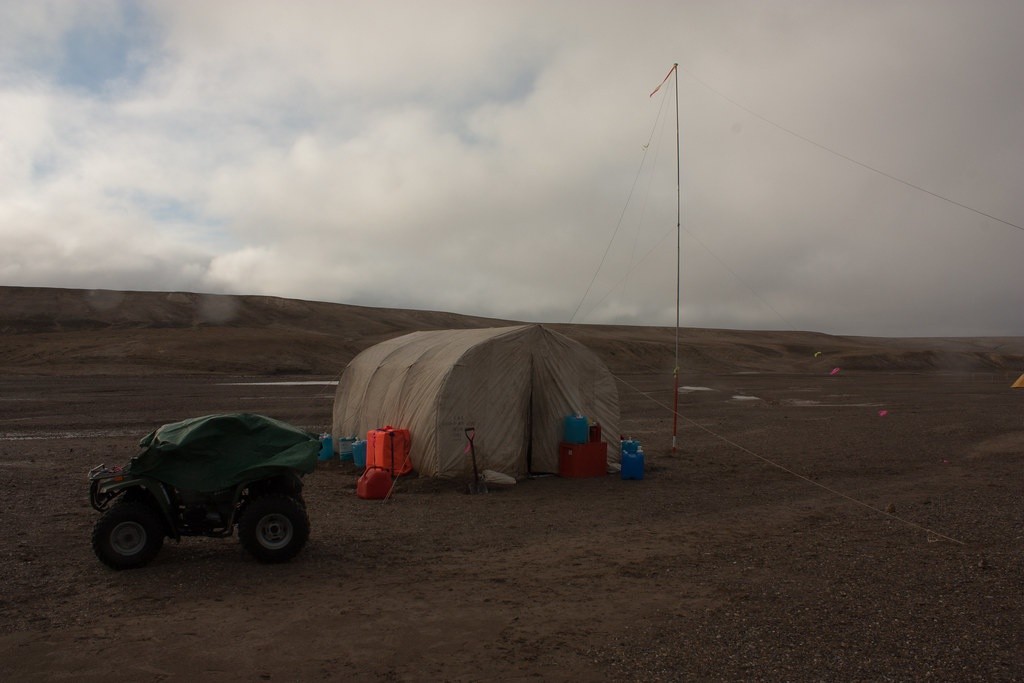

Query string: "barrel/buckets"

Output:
[352,440,366,467]
[318,439,333,459]
[590,422,601,442]
[564,416,588,443]
[358,466,393,499]
[622,450,644,480]
[621,440,640,452]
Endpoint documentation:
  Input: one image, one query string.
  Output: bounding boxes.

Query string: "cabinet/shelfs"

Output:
[559,442,607,479]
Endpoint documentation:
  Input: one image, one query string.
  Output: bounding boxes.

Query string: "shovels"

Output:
[465,424,488,493]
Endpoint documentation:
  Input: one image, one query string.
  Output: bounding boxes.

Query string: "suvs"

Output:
[88,413,323,571]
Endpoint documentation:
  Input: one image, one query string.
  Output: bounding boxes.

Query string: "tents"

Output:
[332,324,624,483]
